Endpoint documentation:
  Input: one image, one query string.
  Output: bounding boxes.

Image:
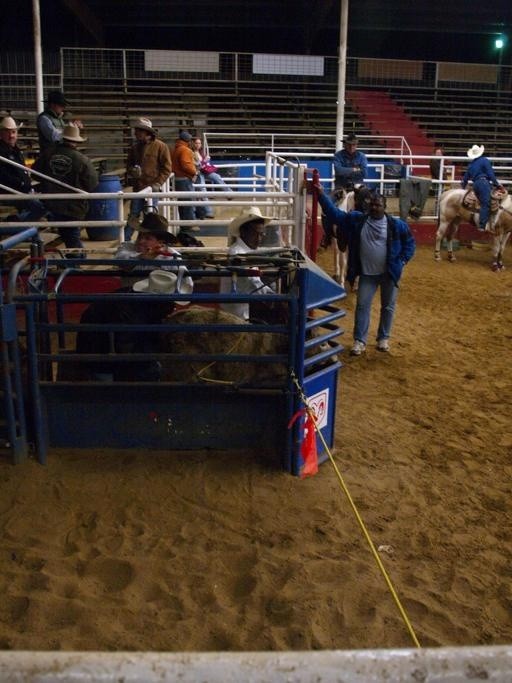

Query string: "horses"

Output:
[331,184,381,290]
[434,188,512,273]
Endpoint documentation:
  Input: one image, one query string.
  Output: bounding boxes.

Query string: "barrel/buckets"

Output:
[84,175,123,242]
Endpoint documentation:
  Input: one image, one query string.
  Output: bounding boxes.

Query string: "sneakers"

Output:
[377,342,391,352]
[350,342,365,356]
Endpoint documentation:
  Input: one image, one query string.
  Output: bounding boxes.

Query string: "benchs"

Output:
[345,81,512,180]
[1,77,337,163]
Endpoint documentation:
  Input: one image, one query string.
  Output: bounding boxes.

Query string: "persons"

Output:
[460,144,505,233]
[430,147,451,225]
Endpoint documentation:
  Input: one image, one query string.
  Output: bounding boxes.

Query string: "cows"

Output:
[159,307,337,383]
[73,285,166,381]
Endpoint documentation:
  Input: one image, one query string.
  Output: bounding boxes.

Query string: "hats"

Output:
[129,117,158,135]
[39,89,68,110]
[126,211,174,241]
[226,205,276,237]
[342,133,361,146]
[60,123,88,144]
[0,117,23,132]
[466,144,484,159]
[130,269,190,305]
[178,131,193,142]
